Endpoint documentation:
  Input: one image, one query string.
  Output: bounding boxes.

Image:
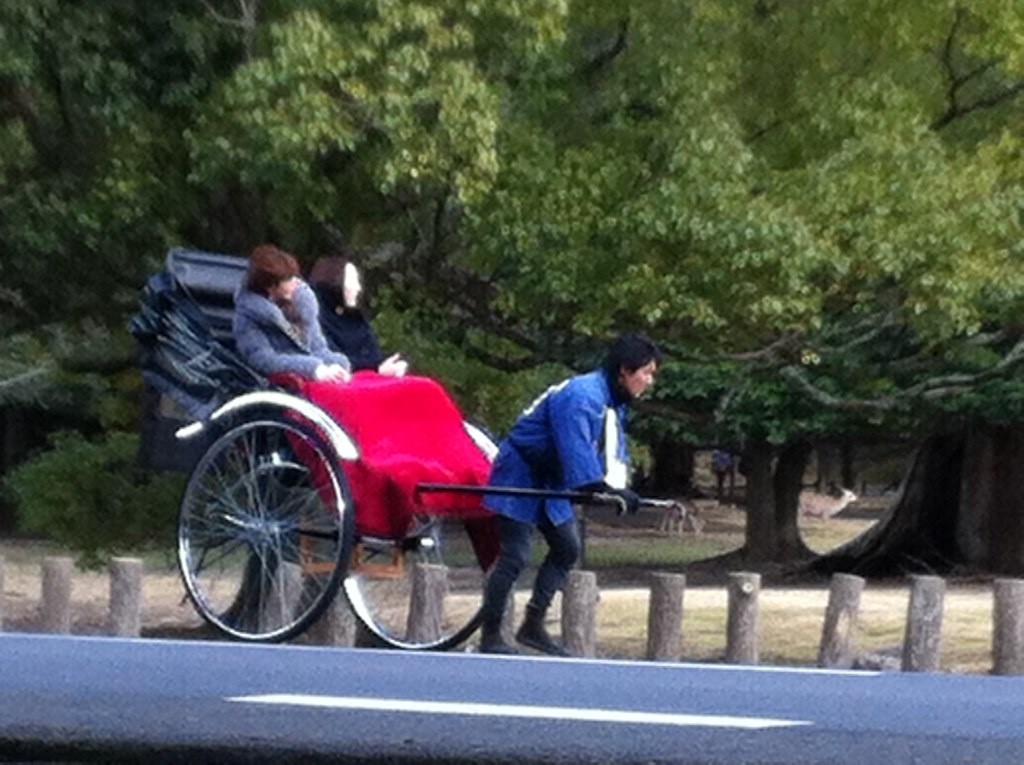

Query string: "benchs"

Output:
[171,303,313,482]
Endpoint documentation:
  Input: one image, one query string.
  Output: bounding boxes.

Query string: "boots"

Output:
[480,617,518,654]
[516,603,571,656]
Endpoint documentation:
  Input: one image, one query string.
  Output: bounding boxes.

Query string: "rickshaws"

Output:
[121,246,701,653]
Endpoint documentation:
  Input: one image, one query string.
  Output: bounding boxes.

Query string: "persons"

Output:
[475,336,662,655]
[233,244,352,387]
[308,255,410,378]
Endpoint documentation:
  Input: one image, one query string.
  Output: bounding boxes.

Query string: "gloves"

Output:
[377,351,409,378]
[313,362,351,384]
[592,484,639,516]
